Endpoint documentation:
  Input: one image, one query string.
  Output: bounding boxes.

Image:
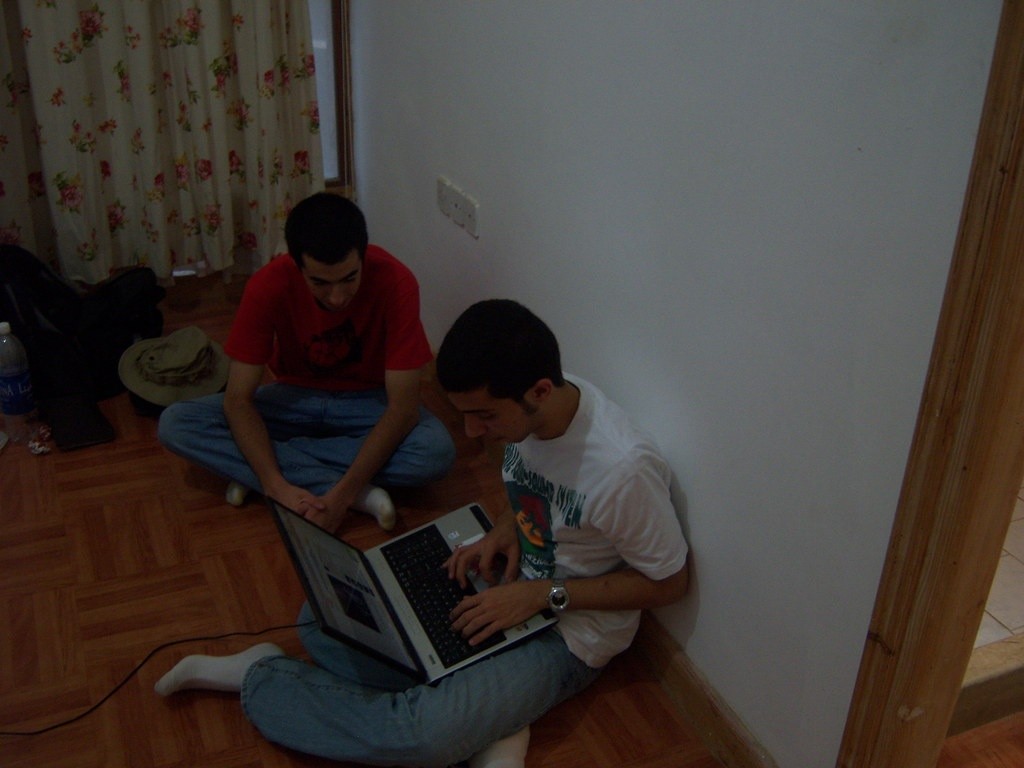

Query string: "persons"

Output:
[155,193,453,533]
[152,299,690,768]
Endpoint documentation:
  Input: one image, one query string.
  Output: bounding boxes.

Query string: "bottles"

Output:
[0,321,37,447]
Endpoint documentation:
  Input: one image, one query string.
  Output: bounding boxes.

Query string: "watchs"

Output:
[546,577,570,611]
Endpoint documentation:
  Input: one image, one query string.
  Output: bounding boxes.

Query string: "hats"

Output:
[117,325,232,408]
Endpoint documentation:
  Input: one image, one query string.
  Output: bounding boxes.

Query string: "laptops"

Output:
[262,493,560,687]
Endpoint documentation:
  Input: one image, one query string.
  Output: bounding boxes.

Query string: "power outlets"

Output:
[463,195,480,240]
[436,175,451,216]
[450,186,466,228]
